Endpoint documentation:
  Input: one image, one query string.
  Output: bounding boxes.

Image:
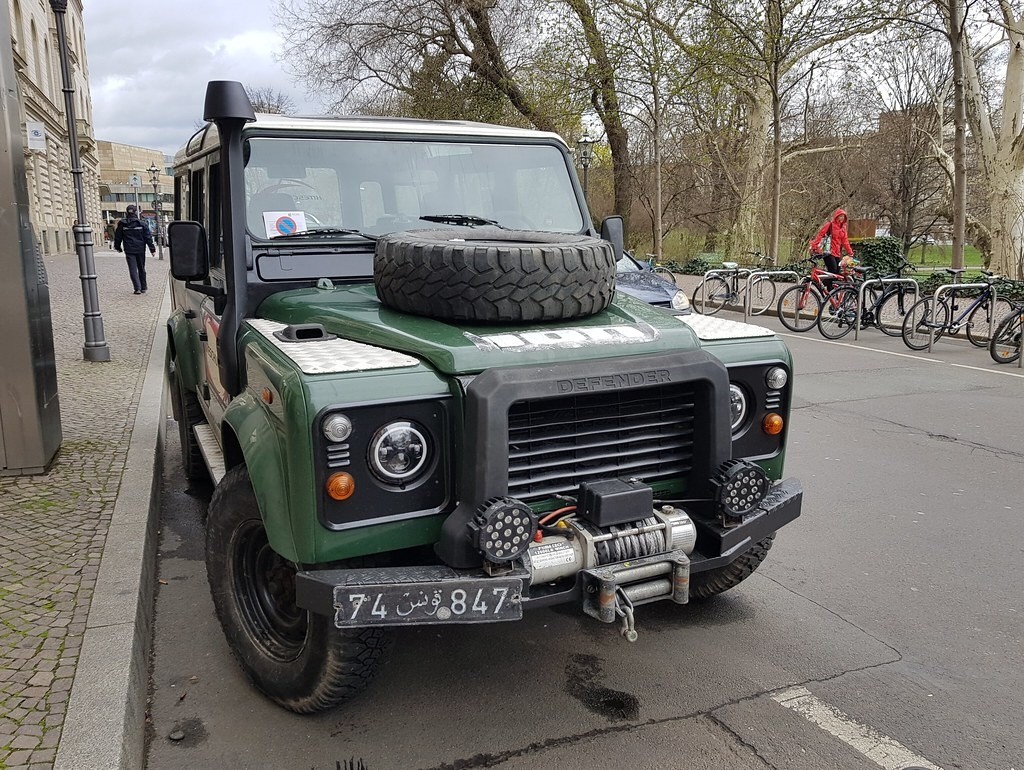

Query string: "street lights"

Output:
[146,161,164,260]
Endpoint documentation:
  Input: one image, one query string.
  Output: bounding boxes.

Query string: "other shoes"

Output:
[134,290,141,294]
[140,289,147,293]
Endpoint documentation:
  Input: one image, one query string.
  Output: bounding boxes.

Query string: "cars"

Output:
[596,233,691,312]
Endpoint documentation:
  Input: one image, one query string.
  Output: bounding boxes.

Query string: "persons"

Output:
[810,207,855,277]
[106,219,118,249]
[72,219,80,255]
[113,203,157,294]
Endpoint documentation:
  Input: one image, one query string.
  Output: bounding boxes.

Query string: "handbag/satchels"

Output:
[811,233,832,258]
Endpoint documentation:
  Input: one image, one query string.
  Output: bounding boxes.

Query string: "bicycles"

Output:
[817,249,929,340]
[627,249,677,286]
[902,268,1014,352]
[990,301,1024,363]
[692,252,776,317]
[778,251,878,333]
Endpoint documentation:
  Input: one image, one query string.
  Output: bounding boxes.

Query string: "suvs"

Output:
[162,81,805,716]
